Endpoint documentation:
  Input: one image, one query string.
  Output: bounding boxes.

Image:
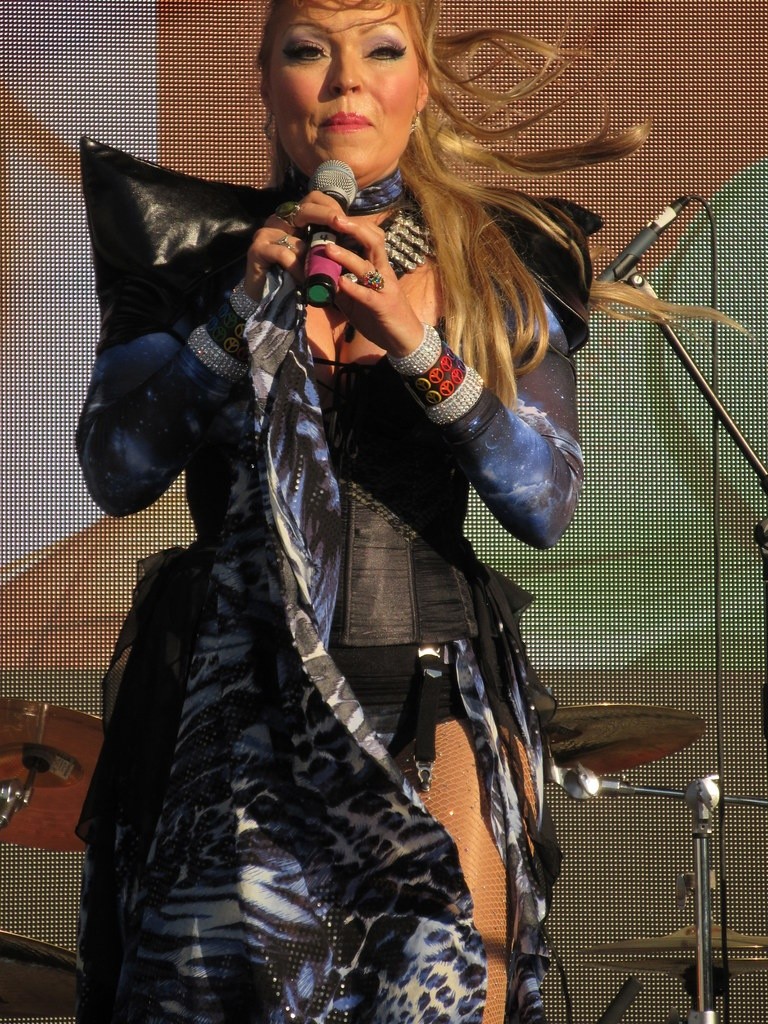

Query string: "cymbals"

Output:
[578,924,768,976]
[536,696,704,781]
[1,693,101,850]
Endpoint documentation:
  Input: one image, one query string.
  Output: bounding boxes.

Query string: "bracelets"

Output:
[386,321,485,426]
[188,279,261,381]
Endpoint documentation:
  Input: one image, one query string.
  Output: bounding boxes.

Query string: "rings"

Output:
[272,201,301,230]
[277,235,294,250]
[361,269,384,291]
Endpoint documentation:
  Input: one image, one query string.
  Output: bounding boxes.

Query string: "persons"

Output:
[74,0,604,1024]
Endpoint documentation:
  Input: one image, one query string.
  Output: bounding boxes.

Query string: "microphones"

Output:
[595,196,692,283]
[303,159,358,308]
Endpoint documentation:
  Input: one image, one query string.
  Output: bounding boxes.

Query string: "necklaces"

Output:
[294,170,406,214]
[333,207,437,343]
